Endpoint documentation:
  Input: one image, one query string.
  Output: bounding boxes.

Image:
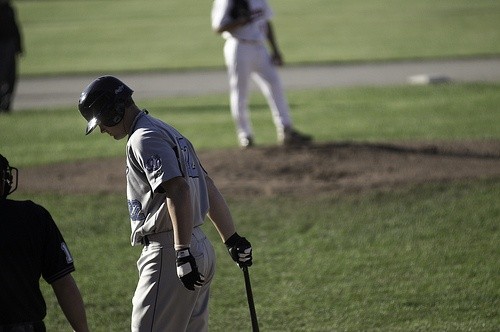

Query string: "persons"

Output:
[78,76,253,332]
[211,0,312,150]
[0,153,90,332]
[0,0,25,115]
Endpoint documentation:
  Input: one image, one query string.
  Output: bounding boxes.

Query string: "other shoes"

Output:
[238,137,253,149]
[279,130,311,144]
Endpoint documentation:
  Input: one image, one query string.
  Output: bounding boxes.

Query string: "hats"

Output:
[79,75,135,136]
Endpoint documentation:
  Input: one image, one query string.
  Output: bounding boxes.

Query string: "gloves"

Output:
[175,244,205,293]
[223,233,252,273]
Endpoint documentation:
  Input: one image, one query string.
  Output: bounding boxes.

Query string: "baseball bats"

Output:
[243,265,261,332]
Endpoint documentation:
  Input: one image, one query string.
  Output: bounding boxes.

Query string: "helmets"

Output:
[0,155,18,199]
